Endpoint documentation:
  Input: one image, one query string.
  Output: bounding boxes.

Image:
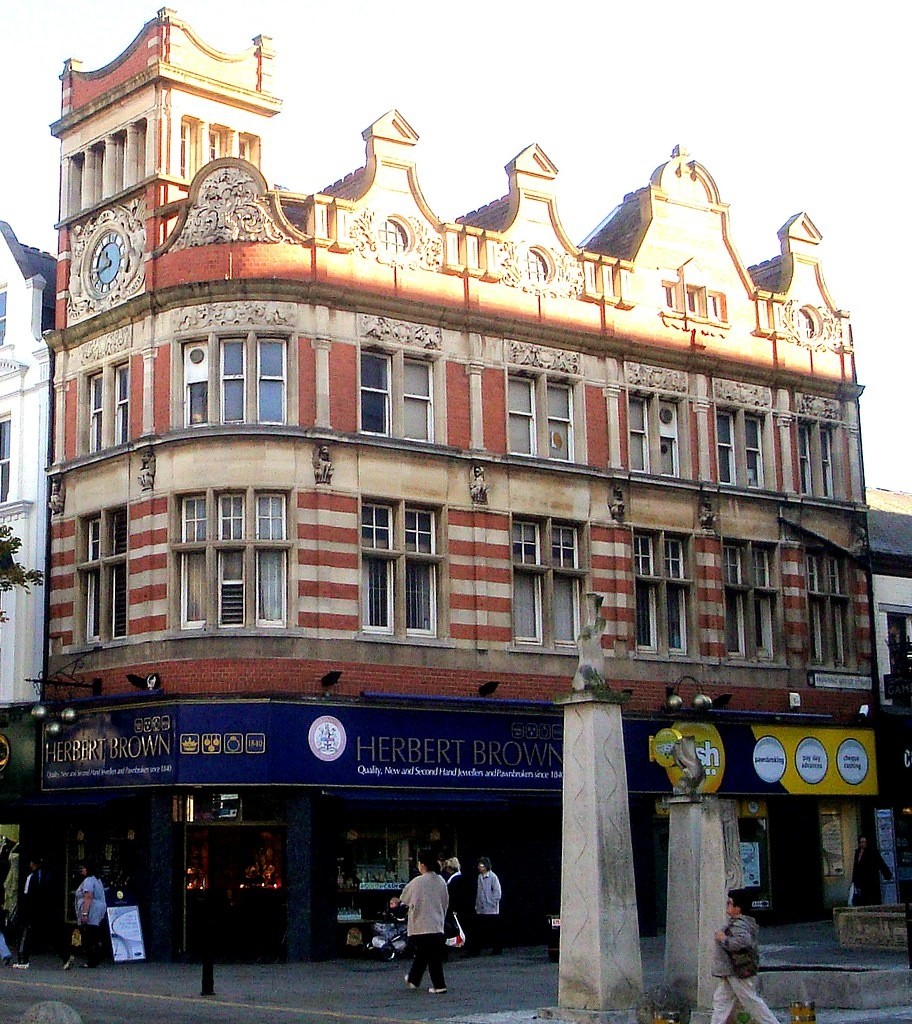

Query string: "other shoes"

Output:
[488,950,501,956]
[79,963,90,967]
[5,956,15,967]
[62,954,74,970]
[12,961,30,969]
[404,974,417,989]
[428,987,447,993]
[480,948,488,957]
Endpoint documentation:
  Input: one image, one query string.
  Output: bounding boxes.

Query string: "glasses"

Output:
[727,901,733,906]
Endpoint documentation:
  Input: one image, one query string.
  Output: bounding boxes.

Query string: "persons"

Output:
[399,855,449,993]
[74,860,107,968]
[710,889,779,1024]
[436,856,467,961]
[12,856,75,969]
[377,897,404,941]
[852,835,892,906]
[475,856,502,956]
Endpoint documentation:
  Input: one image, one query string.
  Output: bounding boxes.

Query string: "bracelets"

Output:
[82,913,88,915]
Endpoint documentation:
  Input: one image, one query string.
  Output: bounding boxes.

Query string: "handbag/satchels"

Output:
[445,915,466,948]
[722,918,759,979]
